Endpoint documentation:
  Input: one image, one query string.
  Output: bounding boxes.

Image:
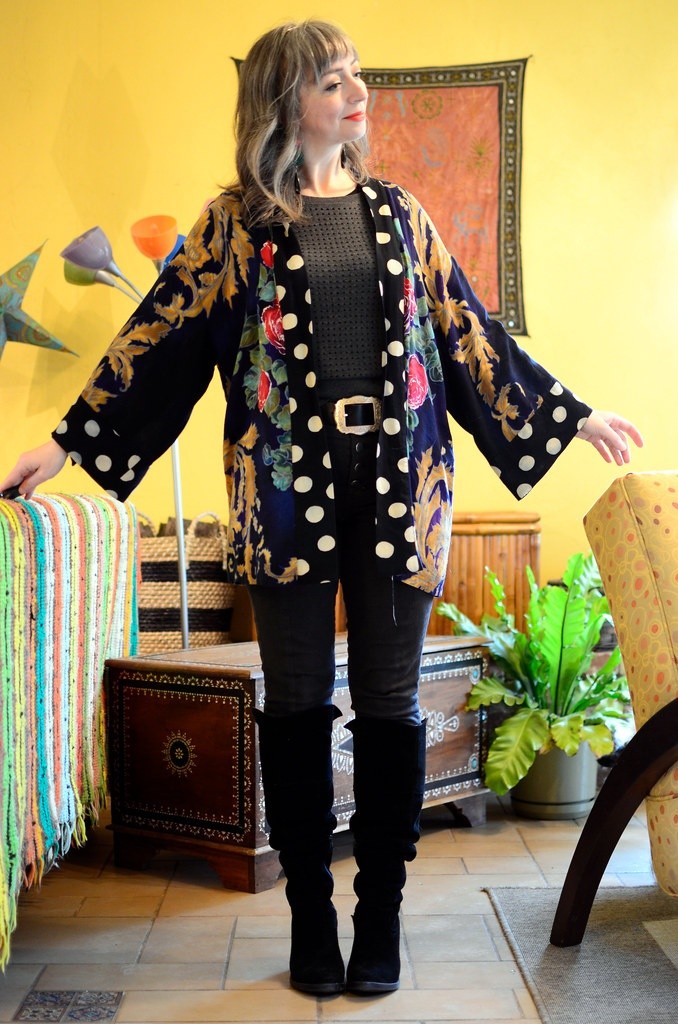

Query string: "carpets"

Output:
[484,883,678,1024]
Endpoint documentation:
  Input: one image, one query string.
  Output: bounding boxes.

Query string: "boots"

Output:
[346,717,428,992]
[246,702,343,996]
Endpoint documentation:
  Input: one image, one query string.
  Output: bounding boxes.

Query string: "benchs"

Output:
[102,633,492,894]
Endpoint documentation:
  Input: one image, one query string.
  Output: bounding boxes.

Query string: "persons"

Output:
[0,21,642,998]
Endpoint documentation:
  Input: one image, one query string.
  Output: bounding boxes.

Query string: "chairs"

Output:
[548,472,676,945]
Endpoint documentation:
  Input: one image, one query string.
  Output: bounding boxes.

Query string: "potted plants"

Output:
[436,554,632,821]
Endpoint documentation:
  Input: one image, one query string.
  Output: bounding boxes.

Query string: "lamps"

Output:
[60,216,192,648]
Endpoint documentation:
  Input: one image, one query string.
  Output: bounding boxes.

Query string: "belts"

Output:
[289,394,397,435]
